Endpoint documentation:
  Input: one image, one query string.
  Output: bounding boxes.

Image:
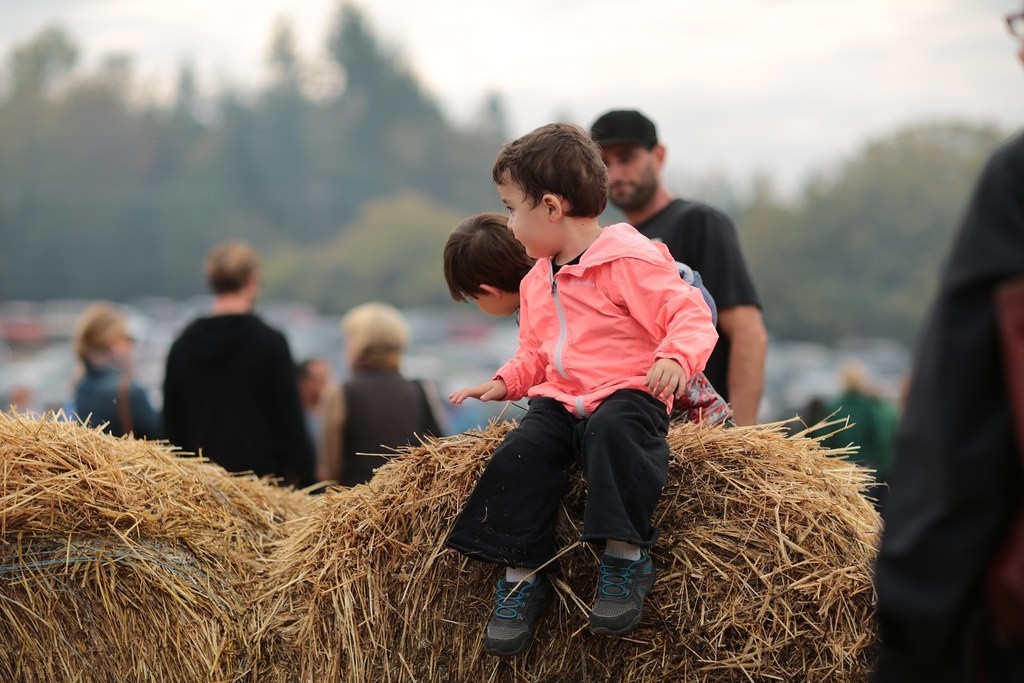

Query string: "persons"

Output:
[293,358,332,477]
[73,304,159,438]
[159,241,322,490]
[441,214,740,432]
[446,120,719,651]
[318,301,444,490]
[589,112,768,431]
[799,364,911,486]
[872,1,1024,682]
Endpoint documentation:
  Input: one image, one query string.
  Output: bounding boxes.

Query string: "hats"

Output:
[591,111,656,150]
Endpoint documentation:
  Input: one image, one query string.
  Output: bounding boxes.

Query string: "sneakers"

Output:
[485,572,553,657]
[589,549,656,633]
